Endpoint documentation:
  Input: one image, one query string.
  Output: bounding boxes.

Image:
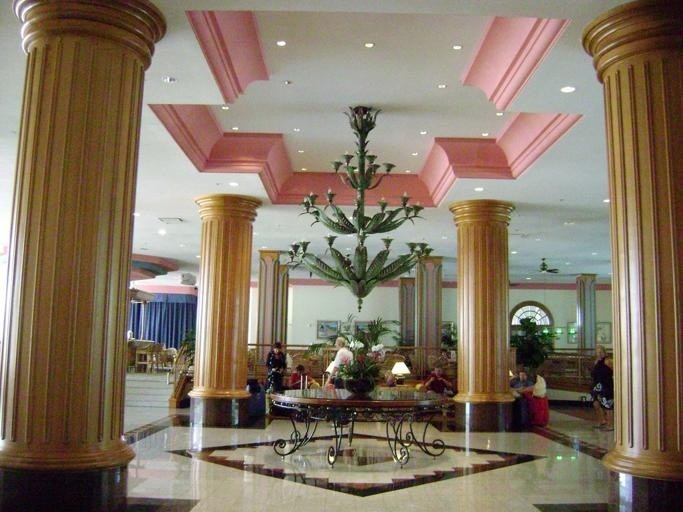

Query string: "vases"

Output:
[345,380,375,394]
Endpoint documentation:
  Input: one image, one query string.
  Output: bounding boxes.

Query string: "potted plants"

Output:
[182,328,196,371]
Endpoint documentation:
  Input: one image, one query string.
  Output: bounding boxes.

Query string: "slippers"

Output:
[593,422,606,428]
[604,427,614,432]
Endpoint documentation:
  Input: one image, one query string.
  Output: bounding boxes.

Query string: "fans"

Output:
[535,258,560,274]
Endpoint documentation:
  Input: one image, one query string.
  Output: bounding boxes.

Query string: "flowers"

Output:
[331,347,383,379]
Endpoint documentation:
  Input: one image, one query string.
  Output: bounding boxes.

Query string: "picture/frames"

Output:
[566,322,578,344]
[595,322,612,344]
[354,320,375,335]
[317,320,341,339]
[442,321,453,340]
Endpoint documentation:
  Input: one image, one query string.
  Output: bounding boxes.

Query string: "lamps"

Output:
[555,327,563,340]
[286,104,434,311]
[542,326,549,336]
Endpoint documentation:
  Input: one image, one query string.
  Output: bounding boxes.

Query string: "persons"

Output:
[325,335,358,385]
[267,342,286,392]
[423,367,451,400]
[588,343,617,431]
[287,364,314,392]
[435,348,450,367]
[509,370,533,388]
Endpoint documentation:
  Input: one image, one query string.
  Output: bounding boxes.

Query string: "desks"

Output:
[270,387,449,469]
